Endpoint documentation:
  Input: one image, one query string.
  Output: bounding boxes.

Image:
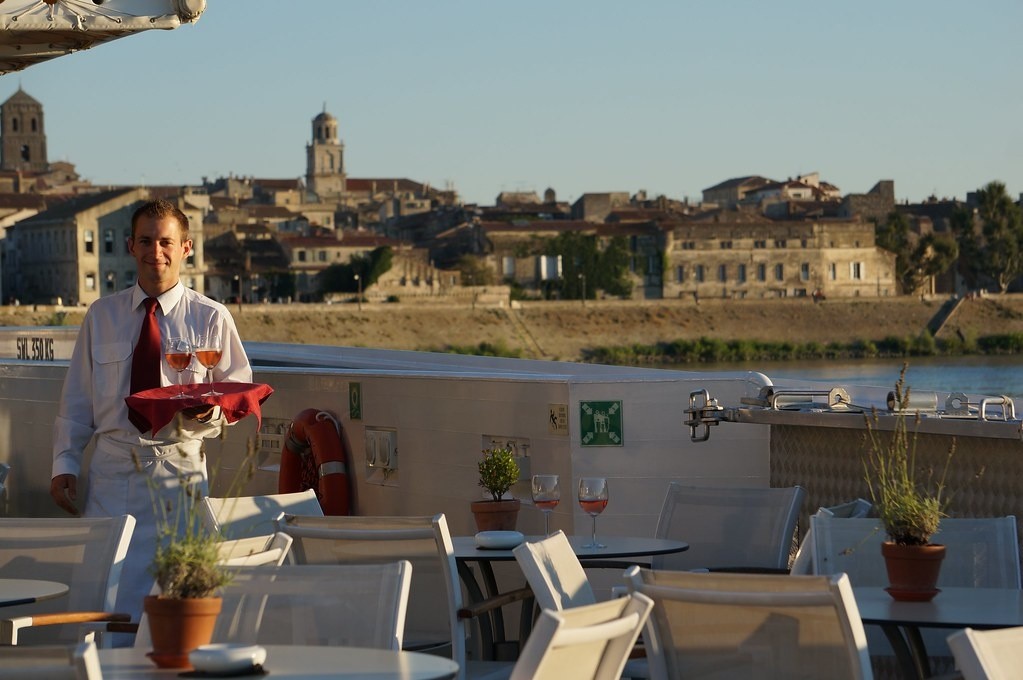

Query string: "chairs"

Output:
[622,479,807,658]
[0,641,104,680]
[790,498,872,575]
[0,513,137,646]
[202,487,331,539]
[207,560,414,650]
[511,528,651,680]
[809,514,1020,590]
[945,623,1023,680]
[271,510,535,680]
[621,563,874,680]
[77,531,293,647]
[507,590,654,680]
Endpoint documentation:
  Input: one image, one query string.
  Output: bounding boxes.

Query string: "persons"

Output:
[47,195,252,647]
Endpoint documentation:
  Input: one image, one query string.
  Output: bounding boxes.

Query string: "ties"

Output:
[128,297,161,434]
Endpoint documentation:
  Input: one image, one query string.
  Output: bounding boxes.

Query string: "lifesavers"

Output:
[279,408,350,518]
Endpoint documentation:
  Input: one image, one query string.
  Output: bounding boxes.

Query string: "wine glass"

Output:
[531,474,560,538]
[578,477,609,548]
[194,333,224,397]
[165,337,193,399]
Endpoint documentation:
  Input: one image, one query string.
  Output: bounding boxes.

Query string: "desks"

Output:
[0,578,70,608]
[97,641,463,680]
[454,534,691,661]
[852,588,1023,680]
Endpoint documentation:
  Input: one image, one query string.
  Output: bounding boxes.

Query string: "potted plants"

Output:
[470,447,520,531]
[129,411,260,668]
[839,357,985,602]
